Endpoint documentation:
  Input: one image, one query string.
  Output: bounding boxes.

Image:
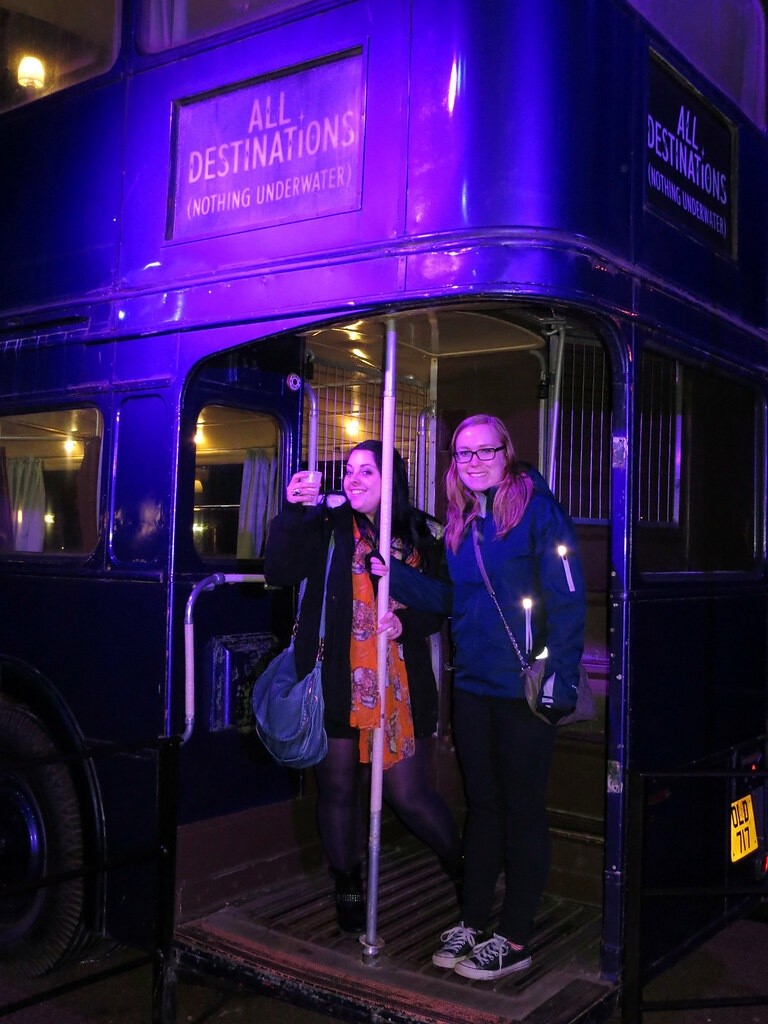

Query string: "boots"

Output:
[456,889,494,919]
[329,859,367,936]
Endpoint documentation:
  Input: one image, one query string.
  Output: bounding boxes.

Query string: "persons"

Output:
[263,440,467,933]
[364,413,585,979]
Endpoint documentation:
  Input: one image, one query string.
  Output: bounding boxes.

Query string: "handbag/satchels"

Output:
[524,657,594,726]
[251,644,328,768]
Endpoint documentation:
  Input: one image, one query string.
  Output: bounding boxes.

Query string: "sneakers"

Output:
[454,933,532,981]
[432,921,487,967]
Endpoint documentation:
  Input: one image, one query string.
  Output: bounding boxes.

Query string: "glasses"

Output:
[451,446,508,463]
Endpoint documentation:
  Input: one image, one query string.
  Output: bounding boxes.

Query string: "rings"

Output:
[293,489,300,495]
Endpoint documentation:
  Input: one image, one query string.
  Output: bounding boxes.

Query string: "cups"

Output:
[298,471,322,506]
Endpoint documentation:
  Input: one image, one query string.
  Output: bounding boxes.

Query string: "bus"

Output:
[1,0,768,1024]
